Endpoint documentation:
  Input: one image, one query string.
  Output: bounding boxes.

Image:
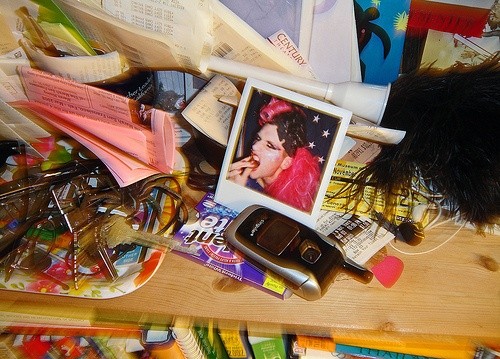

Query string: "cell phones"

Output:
[222,203,374,303]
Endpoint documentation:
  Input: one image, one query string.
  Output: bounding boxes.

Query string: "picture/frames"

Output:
[213,74,353,232]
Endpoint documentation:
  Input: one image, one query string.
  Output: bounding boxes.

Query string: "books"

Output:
[1,301,434,359]
[220,0,316,63]
[212,1,322,86]
[169,190,293,300]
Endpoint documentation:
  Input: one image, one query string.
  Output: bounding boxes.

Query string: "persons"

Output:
[227,99,320,211]
